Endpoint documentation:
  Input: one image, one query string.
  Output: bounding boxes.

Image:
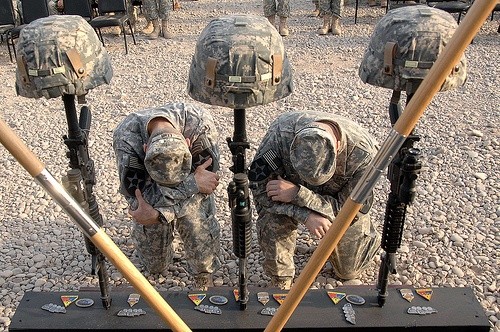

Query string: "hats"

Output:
[289,124,337,186]
[144,133,193,189]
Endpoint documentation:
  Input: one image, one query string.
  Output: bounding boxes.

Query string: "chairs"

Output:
[427,0,475,44]
[0,0,142,64]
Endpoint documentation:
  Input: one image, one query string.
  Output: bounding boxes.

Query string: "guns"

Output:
[226,109,252,312]
[62,94,112,310]
[377,89,422,307]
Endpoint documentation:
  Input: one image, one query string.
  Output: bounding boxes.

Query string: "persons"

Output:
[0,0,172,40]
[248,110,382,290]
[111,103,220,288]
[263,0,472,36]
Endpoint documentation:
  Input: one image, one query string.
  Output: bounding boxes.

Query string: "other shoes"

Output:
[368,0,376,6]
[278,16,288,36]
[111,26,121,35]
[268,16,276,29]
[311,9,319,16]
[380,0,389,7]
[332,16,341,35]
[125,26,135,34]
[149,19,161,40]
[268,276,292,290]
[161,19,174,39]
[193,276,210,291]
[141,20,153,34]
[317,17,331,35]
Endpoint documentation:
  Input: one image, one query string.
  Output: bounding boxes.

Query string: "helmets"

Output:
[13,14,113,101]
[185,14,294,109]
[357,6,469,92]
